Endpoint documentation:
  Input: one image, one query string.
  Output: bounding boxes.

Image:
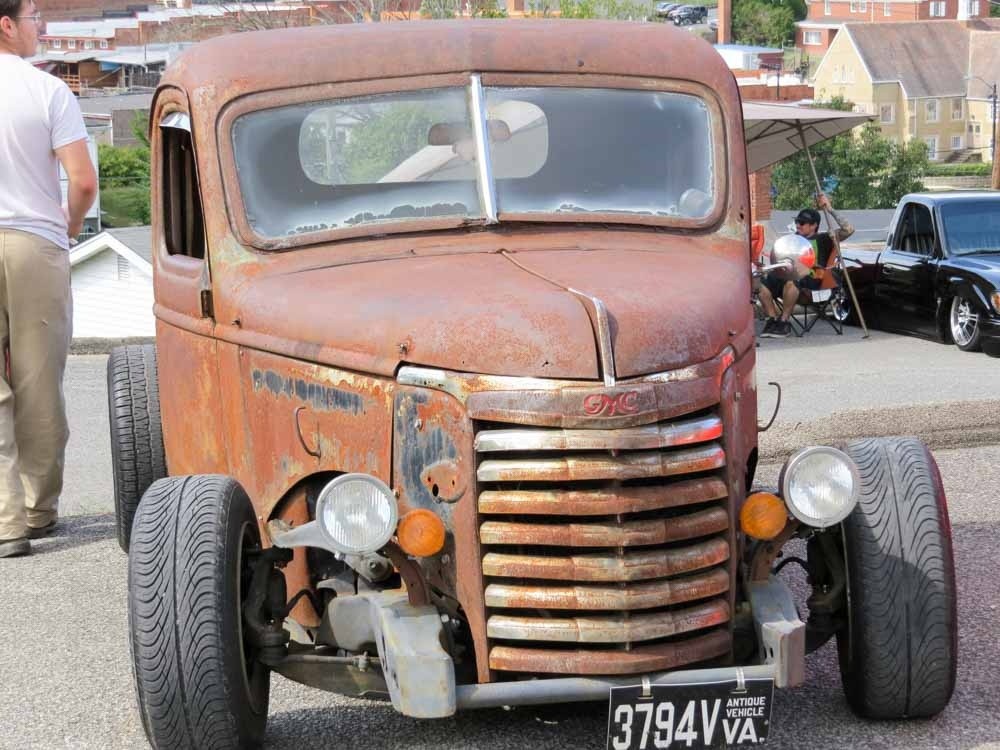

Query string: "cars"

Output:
[104,15,959,750]
[654,2,708,26]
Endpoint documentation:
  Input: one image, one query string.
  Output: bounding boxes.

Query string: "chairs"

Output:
[777,239,844,335]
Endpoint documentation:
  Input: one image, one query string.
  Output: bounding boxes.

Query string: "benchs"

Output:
[901,229,1000,259]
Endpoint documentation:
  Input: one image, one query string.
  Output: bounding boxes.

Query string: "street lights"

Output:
[963,74,997,166]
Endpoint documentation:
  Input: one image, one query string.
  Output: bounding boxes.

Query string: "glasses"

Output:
[795,220,807,225]
[10,13,41,23]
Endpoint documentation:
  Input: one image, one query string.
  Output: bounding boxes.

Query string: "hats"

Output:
[792,209,821,223]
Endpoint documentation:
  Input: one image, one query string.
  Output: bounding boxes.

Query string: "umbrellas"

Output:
[743,100,880,336]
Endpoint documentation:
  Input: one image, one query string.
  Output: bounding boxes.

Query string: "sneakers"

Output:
[0,537,30,558]
[25,520,57,538]
[760,317,776,337]
[770,320,792,337]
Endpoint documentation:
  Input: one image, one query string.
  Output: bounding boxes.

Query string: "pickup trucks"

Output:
[829,191,1000,359]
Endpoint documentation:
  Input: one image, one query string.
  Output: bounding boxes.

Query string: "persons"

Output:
[0,0,99,558]
[757,192,855,337]
